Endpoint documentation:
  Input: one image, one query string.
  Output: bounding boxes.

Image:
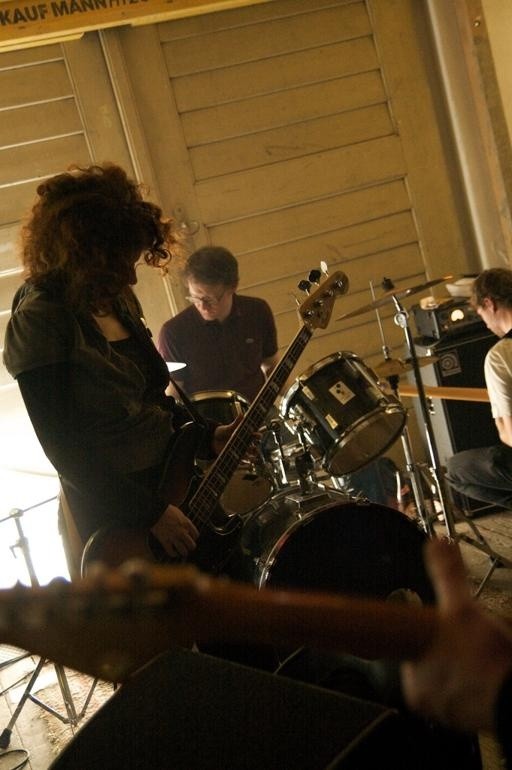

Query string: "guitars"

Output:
[80,262,350,583]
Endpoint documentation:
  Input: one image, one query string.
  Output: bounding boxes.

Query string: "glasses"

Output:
[185,289,226,304]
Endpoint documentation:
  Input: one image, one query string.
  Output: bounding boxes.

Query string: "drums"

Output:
[175,388,275,517]
[235,481,442,608]
[279,350,410,477]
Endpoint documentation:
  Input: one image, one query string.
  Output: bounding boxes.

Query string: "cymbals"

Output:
[372,355,441,376]
[334,274,454,320]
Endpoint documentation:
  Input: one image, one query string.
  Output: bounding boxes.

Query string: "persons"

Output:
[445,265,511,517]
[156,244,298,516]
[4,162,262,671]
[392,534,510,761]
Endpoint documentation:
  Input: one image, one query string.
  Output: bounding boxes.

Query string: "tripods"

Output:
[0,516,118,749]
[389,328,511,599]
